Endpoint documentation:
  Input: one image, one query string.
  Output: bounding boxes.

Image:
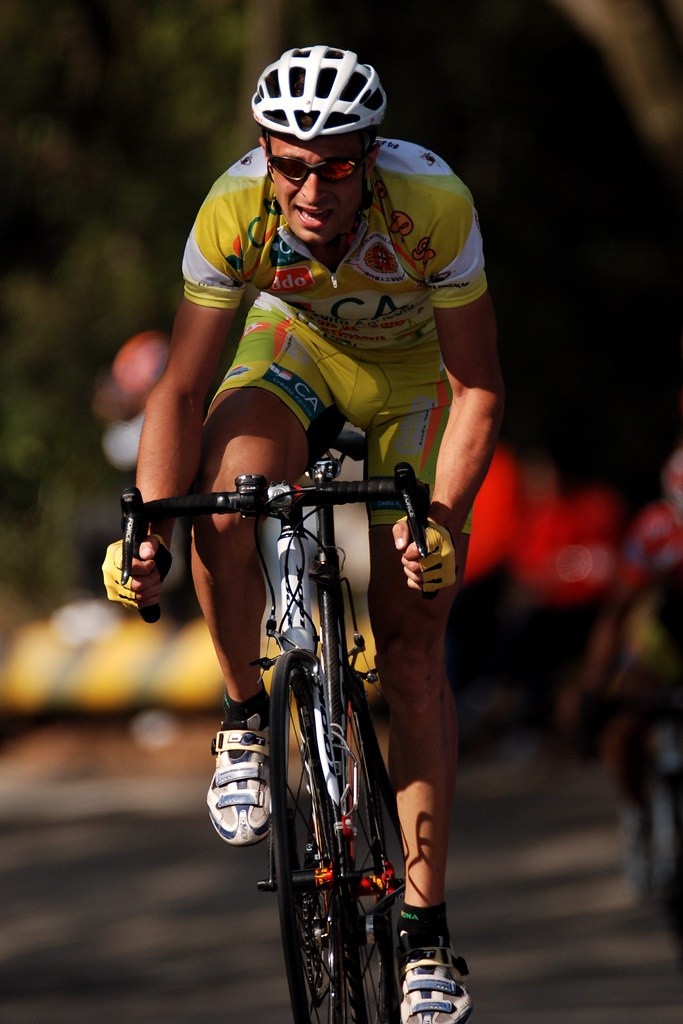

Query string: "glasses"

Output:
[262,132,374,183]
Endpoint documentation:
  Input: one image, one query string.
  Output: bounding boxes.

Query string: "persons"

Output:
[101,45,503,1024]
[555,445,683,841]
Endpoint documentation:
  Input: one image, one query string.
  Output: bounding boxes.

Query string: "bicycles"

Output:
[116,427,443,1024]
[574,689,683,974]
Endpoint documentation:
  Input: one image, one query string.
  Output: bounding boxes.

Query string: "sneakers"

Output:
[206,696,271,845]
[395,932,473,1024]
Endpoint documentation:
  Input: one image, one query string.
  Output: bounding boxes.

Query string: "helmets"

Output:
[251,46,388,141]
